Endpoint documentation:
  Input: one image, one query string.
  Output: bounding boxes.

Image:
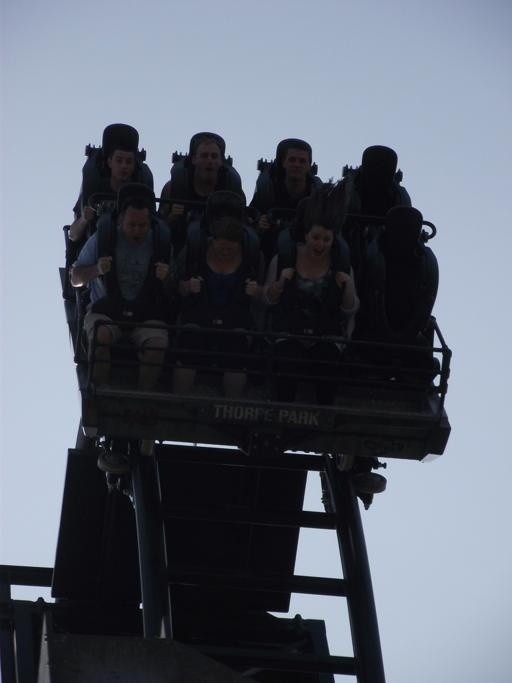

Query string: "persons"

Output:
[67,141,150,244]
[158,136,246,226]
[68,195,174,395]
[261,214,360,406]
[248,140,323,240]
[175,219,259,399]
